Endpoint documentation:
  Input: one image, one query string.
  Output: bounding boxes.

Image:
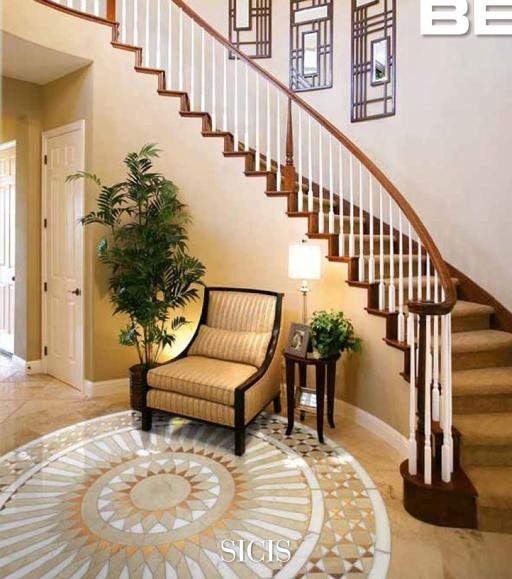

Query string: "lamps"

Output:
[288,244,320,326]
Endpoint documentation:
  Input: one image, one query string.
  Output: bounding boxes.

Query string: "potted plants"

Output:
[308,311,360,360]
[65,141,206,411]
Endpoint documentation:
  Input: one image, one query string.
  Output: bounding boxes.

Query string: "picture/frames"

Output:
[285,323,312,359]
[293,384,319,415]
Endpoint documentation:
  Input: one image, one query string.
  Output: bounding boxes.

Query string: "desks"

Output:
[280,352,341,445]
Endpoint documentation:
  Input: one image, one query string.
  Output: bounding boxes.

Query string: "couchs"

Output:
[140,286,283,457]
